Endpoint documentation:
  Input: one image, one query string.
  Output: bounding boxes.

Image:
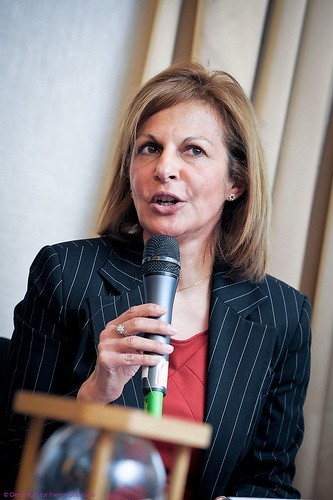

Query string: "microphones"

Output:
[141,235,181,415]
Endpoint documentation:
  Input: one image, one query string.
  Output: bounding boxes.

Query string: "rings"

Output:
[115,324,127,338]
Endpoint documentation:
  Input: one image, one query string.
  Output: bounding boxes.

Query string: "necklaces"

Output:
[175,272,210,293]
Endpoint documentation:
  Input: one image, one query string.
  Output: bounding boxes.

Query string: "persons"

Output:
[0,61,312,500]
